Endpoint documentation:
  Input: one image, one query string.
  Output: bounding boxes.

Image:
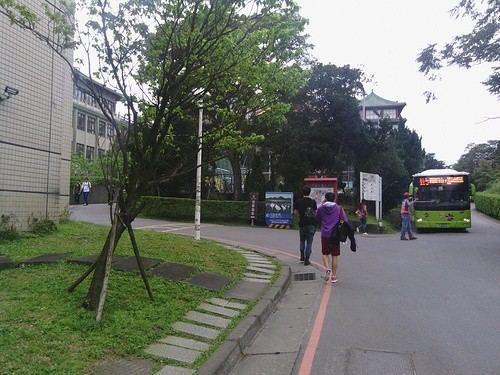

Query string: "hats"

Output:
[404,192,411,196]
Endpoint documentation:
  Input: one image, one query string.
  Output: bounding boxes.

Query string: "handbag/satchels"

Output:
[338,206,347,243]
[356,210,362,219]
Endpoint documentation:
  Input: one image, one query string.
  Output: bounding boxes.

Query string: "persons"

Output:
[73,180,81,204]
[400,192,417,240]
[294,185,318,265]
[355,203,368,235]
[316,193,349,283]
[78,177,92,206]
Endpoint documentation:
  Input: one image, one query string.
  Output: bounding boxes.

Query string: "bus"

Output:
[409,169,475,232]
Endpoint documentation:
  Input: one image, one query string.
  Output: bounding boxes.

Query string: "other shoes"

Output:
[324,268,331,281]
[409,237,417,240]
[330,277,337,283]
[299,256,304,261]
[304,261,310,266]
[363,232,368,235]
[356,228,359,233]
[401,237,408,240]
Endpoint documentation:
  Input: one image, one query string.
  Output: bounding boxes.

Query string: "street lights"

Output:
[187,88,212,241]
